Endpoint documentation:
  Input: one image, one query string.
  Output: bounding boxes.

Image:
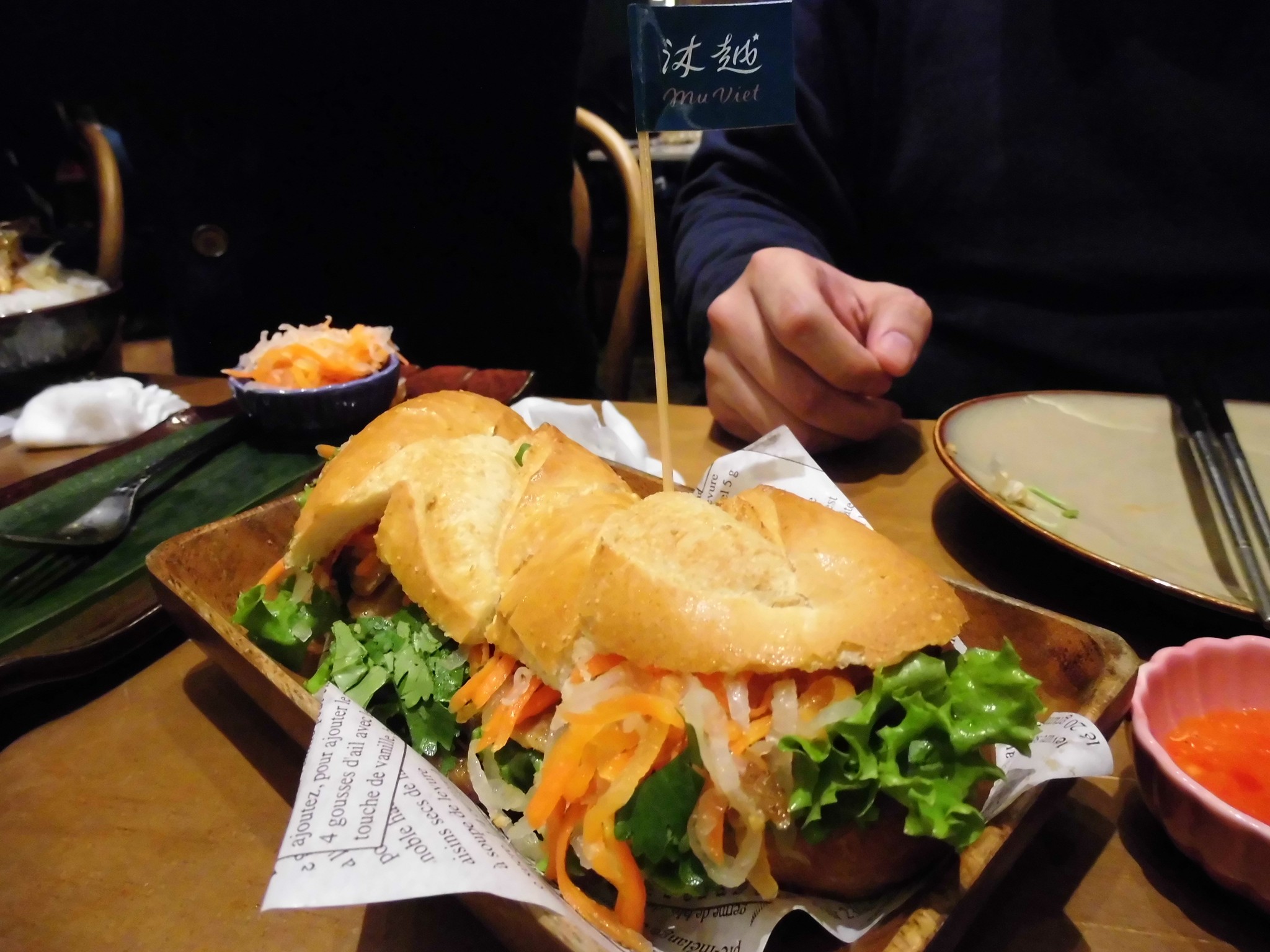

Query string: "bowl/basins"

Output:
[0,279,126,384]
[148,456,1141,952]
[229,351,403,447]
[1125,633,1269,910]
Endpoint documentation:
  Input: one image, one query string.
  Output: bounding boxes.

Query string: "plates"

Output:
[934,388,1269,614]
[0,363,536,694]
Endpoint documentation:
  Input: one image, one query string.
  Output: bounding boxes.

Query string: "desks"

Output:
[0,373,1270,952]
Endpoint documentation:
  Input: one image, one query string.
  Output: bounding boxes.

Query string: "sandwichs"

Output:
[229,385,1048,947]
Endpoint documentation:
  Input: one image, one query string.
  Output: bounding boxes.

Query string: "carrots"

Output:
[219,322,388,391]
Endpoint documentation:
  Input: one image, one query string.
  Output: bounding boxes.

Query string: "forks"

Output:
[0,419,243,552]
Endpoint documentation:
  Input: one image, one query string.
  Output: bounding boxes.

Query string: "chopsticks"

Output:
[1172,382,1269,620]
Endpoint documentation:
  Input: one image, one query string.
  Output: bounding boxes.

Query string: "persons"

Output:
[1,0,659,398]
[670,0,1269,447]
